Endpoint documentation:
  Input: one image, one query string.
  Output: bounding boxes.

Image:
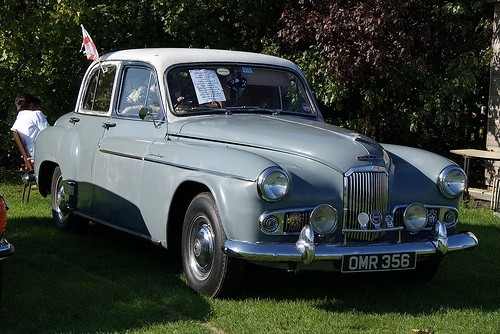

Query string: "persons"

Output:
[8,93,52,171]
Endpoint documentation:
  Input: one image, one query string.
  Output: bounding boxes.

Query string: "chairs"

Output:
[11,128,36,205]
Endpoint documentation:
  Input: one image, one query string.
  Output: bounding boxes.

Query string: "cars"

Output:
[34,47,479,299]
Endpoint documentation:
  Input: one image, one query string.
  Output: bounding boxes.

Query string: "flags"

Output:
[78,23,99,62]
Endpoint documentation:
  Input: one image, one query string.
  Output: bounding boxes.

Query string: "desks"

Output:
[450,148,500,208]
